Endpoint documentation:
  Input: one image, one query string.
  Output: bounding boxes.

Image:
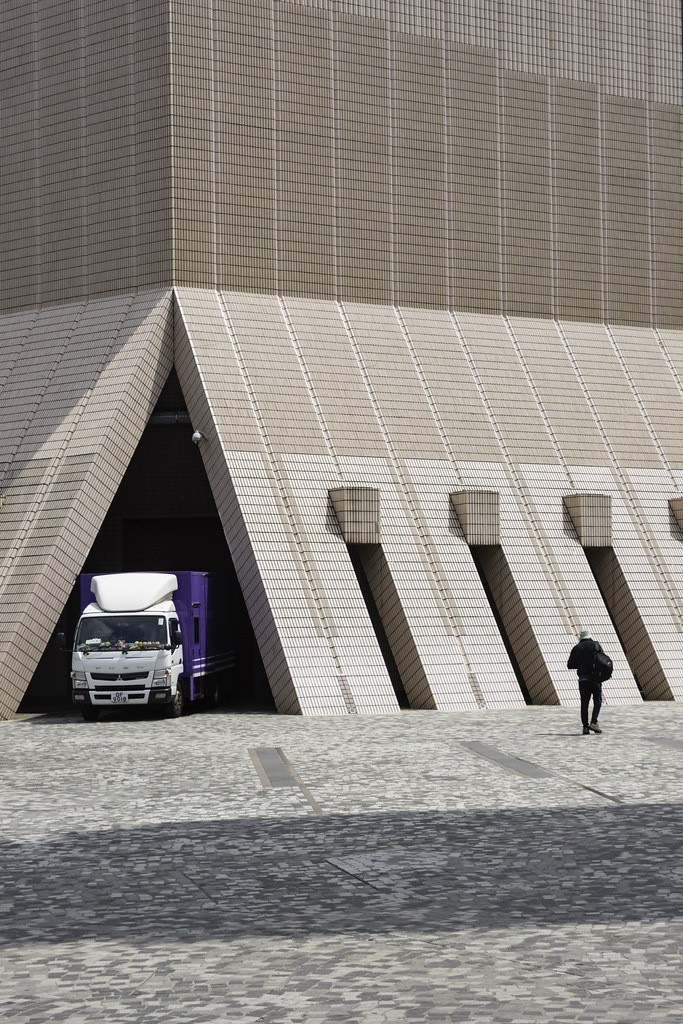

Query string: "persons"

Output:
[567,630,603,734]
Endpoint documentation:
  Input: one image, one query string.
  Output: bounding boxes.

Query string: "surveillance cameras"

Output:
[192,432,202,443]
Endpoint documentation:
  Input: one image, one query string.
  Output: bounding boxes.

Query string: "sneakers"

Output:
[588,722,602,733]
[583,727,590,734]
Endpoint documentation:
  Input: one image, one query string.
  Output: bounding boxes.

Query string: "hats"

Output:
[579,631,591,640]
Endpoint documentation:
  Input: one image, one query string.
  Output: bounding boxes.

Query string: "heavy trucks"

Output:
[54,570,223,721]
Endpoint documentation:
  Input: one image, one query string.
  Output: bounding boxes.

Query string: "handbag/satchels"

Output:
[591,641,613,683]
